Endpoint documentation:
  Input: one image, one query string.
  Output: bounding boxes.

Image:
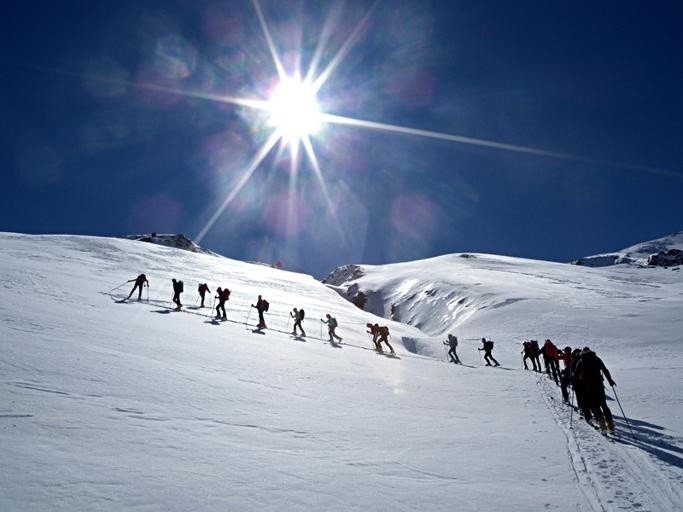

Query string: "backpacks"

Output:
[178,280,184,292]
[484,341,494,350]
[298,308,305,320]
[581,351,604,388]
[222,287,231,301]
[382,326,390,336]
[544,342,556,358]
[262,299,269,312]
[197,283,204,292]
[331,318,338,327]
[451,335,458,347]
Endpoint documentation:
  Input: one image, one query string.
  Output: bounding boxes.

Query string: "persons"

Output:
[477,338,500,367]
[442,334,459,363]
[320,314,342,342]
[250,295,267,328]
[214,287,227,321]
[289,308,306,337]
[374,324,394,353]
[519,338,617,436]
[366,322,378,348]
[170,278,183,311]
[125,273,148,301]
[197,283,211,307]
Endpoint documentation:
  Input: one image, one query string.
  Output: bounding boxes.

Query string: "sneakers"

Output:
[492,363,500,367]
[596,420,606,431]
[606,422,616,430]
[214,314,221,319]
[450,359,460,364]
[255,323,267,330]
[338,338,343,343]
[300,334,306,337]
[484,363,492,366]
[290,332,296,334]
[173,303,183,311]
[220,317,227,322]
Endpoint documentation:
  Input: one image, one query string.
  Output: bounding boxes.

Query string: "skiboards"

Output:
[549,396,584,416]
[580,416,619,443]
